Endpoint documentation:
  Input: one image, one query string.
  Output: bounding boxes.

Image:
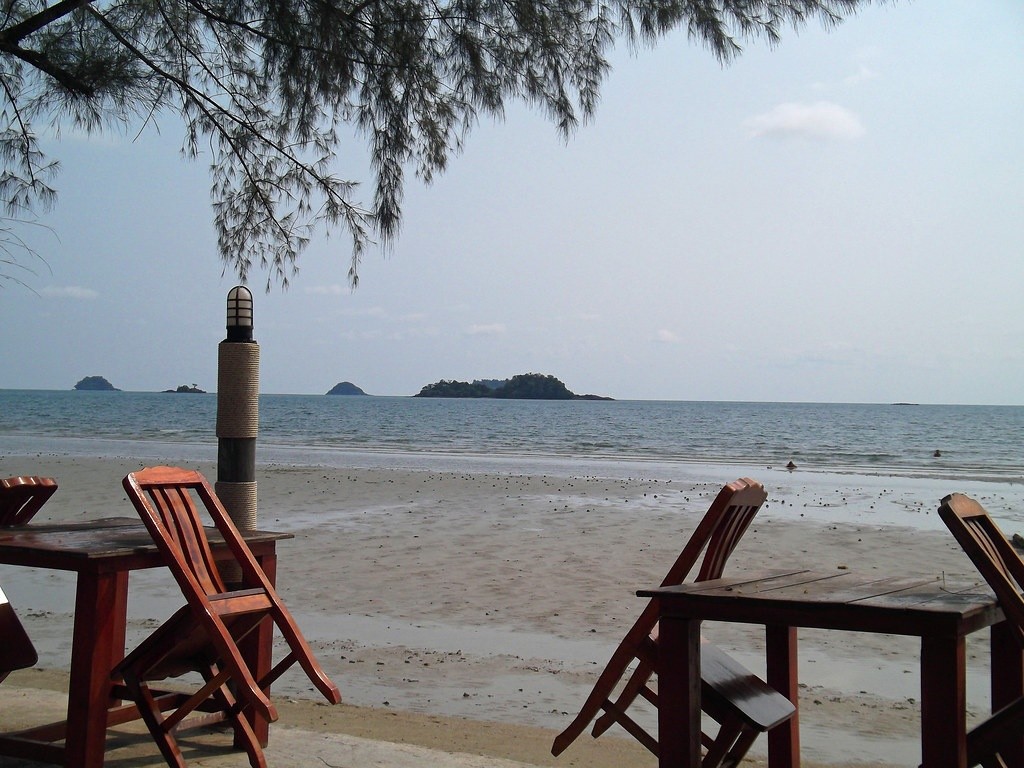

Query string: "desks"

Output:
[632,566,1023,767]
[0,512,293,767]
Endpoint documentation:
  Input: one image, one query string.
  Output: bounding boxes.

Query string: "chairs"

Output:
[0,475,58,684]
[108,466,342,768]
[551,475,797,768]
[910,492,1024,768]
